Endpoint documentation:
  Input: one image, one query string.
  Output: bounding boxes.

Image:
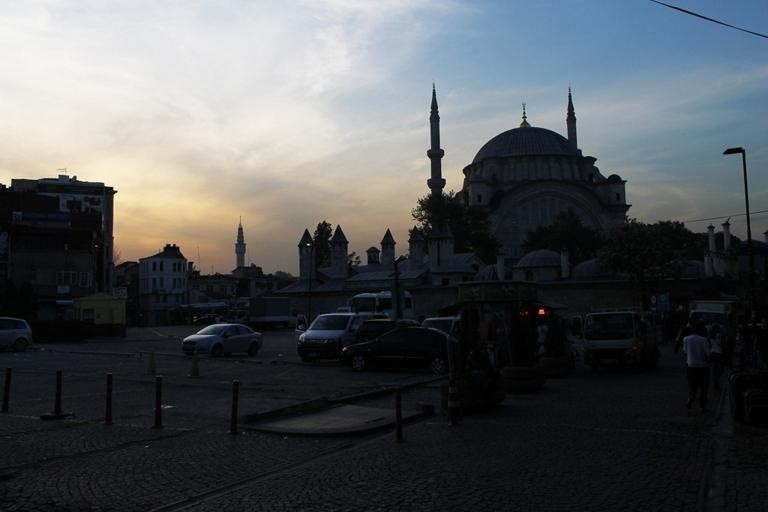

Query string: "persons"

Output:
[672,301,767,413]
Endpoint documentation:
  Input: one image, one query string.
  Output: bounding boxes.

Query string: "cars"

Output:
[193,313,224,325]
[182,323,264,358]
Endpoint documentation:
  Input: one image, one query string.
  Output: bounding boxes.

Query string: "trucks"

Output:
[227,307,249,325]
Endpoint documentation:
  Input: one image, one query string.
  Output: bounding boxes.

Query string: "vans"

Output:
[0,318,34,352]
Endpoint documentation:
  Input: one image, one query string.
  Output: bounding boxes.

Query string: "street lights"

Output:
[721,146,757,325]
